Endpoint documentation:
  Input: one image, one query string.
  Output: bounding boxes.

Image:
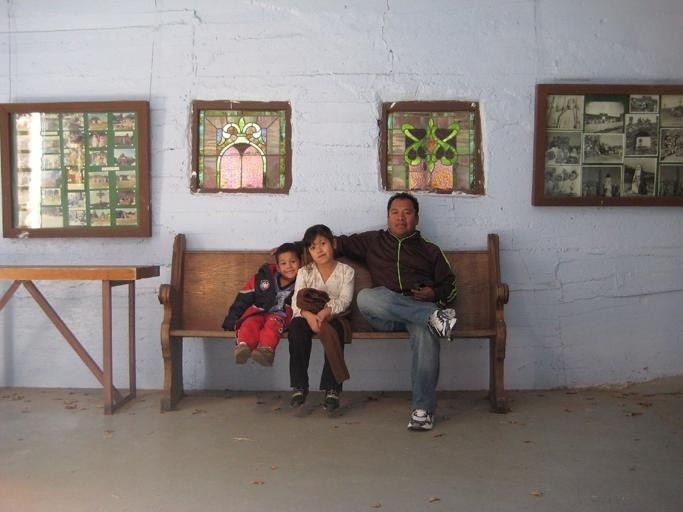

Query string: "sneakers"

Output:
[288,386,309,408]
[406,408,433,430]
[427,308,458,343]
[250,346,274,366]
[232,342,251,364]
[322,388,339,411]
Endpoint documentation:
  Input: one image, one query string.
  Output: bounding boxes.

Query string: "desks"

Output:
[0,263,160,416]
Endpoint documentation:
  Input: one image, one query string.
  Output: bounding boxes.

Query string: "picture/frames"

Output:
[0,100,152,238]
[532,84,683,207]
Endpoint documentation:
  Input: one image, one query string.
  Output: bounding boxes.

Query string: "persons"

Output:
[545,93,682,195]
[220,242,302,368]
[287,222,357,414]
[268,188,461,430]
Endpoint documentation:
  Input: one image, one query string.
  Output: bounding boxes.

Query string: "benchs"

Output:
[158,234,512,413]
